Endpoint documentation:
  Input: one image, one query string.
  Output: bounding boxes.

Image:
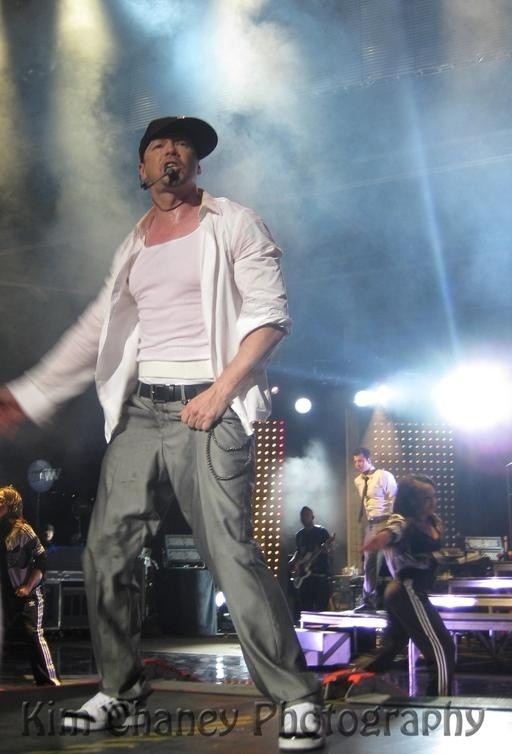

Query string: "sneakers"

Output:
[61,692,148,731]
[354,604,376,614]
[278,702,325,750]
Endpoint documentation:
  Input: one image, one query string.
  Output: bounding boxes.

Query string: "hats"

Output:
[138,116,219,164]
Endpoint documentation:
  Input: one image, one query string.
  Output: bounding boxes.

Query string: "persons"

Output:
[0,485,62,688]
[289,506,336,613]
[358,471,457,697]
[38,524,61,558]
[0,114,328,751]
[350,447,398,612]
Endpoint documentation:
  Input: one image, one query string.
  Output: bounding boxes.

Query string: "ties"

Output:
[358,475,369,522]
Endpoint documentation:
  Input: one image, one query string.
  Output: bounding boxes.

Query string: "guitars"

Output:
[293,532,335,590]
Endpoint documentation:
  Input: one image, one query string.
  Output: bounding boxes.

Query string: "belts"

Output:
[133,380,212,403]
[369,516,388,524]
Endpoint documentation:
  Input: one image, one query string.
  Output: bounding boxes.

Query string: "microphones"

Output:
[139,167,174,190]
